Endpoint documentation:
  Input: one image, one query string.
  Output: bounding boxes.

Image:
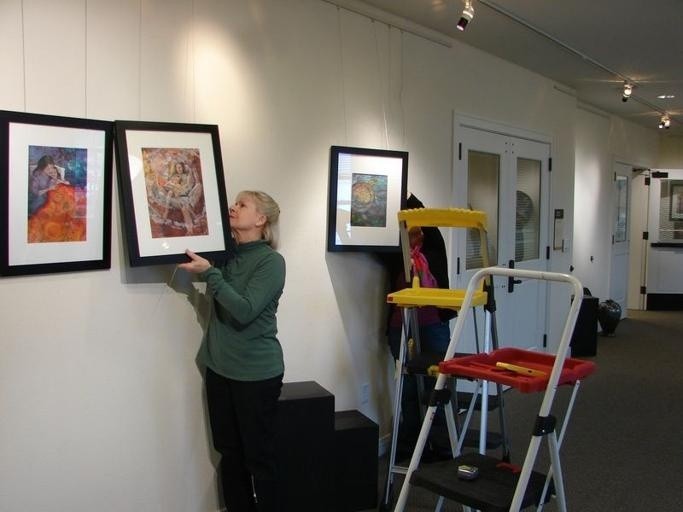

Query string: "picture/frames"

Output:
[327,146,408,253]
[0,109,112,279]
[114,119,234,268]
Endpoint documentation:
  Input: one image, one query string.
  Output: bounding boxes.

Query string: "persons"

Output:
[30,155,71,214]
[373,190,466,460]
[177,190,286,512]
[161,161,195,235]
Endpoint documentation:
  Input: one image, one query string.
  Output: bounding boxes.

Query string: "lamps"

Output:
[456,5,474,31]
[621,83,633,102]
[670,183,683,220]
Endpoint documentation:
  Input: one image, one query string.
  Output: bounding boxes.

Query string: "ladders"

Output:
[397,268,595,511]
[384,205,512,511]
